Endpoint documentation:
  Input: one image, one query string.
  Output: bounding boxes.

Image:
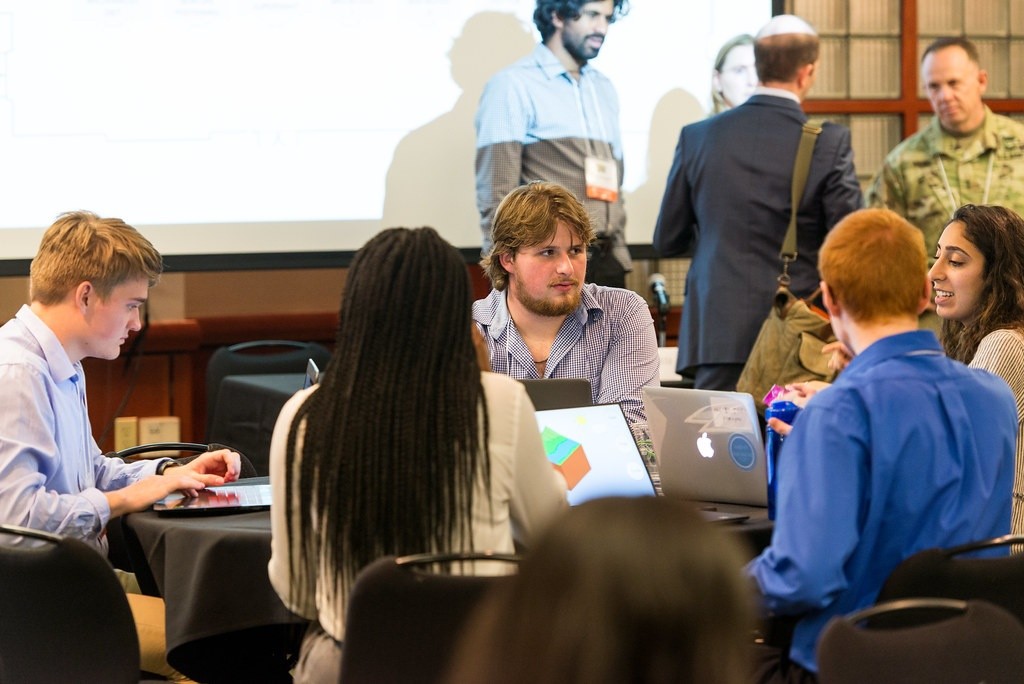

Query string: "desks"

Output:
[124,475,776,684]
[210,347,693,476]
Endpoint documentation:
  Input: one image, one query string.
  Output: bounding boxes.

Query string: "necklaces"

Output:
[534,358,548,364]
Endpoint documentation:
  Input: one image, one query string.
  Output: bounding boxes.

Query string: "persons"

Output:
[267,225,570,684]
[737,203,1023,684]
[863,35,1024,338]
[472,179,661,472]
[707,33,761,125]
[0,210,241,684]
[473,0,635,292]
[445,492,777,684]
[651,13,870,450]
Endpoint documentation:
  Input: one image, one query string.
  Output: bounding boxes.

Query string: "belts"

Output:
[586,238,612,259]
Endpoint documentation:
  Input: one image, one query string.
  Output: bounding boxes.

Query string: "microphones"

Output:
[648,272,670,315]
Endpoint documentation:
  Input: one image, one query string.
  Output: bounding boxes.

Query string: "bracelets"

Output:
[158,462,180,475]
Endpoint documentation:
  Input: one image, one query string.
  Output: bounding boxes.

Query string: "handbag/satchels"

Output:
[736,271,843,417]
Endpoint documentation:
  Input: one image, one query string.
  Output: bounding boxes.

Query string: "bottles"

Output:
[764,396,802,520]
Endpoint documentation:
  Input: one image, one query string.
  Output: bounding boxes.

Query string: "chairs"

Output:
[0,340,1024,684]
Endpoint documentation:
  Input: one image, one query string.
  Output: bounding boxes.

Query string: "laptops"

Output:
[515,378,773,527]
[151,358,319,517]
